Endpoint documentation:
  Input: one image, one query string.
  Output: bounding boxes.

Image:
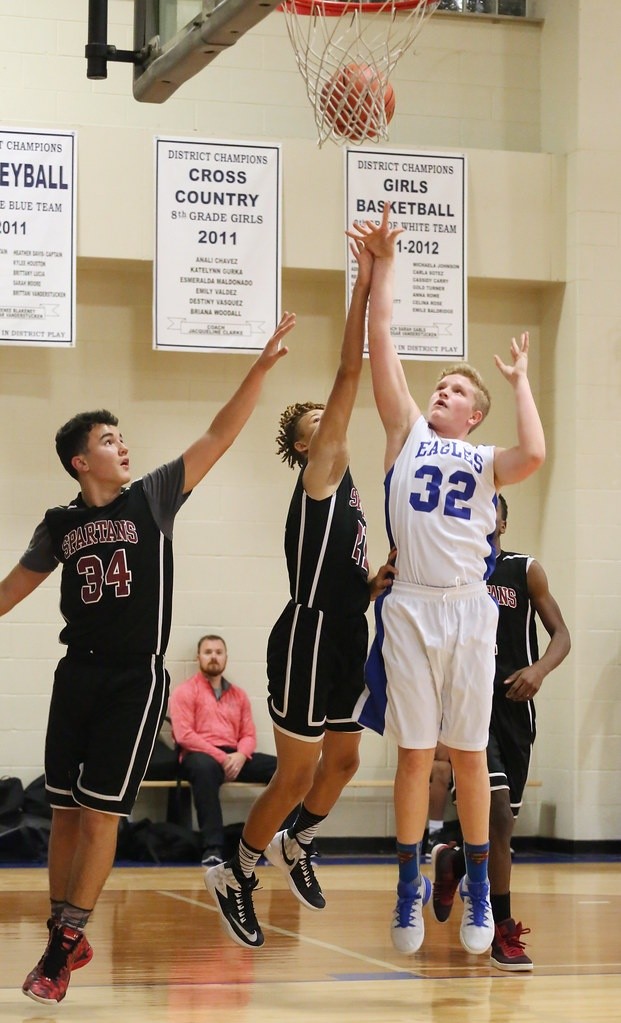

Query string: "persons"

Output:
[204,235,397,948]
[424,743,457,858]
[421,493,571,971]
[0,310,297,1004]
[345,200,547,954]
[166,633,322,864]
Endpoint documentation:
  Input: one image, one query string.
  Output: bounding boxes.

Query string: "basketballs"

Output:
[319,61,397,143]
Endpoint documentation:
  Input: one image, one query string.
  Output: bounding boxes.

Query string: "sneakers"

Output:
[390,876,435,956]
[491,918,535,971]
[47,918,93,970]
[429,841,461,923]
[265,829,325,910]
[457,874,493,953]
[208,855,264,949]
[24,925,86,1005]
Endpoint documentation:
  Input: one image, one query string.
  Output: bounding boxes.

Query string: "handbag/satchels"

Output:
[0,777,24,823]
[24,774,52,817]
[144,716,177,781]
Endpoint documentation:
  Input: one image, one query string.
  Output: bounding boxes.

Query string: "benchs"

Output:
[142,777,542,833]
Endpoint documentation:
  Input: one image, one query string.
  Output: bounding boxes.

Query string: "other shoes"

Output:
[426,840,432,857]
[203,848,222,864]
